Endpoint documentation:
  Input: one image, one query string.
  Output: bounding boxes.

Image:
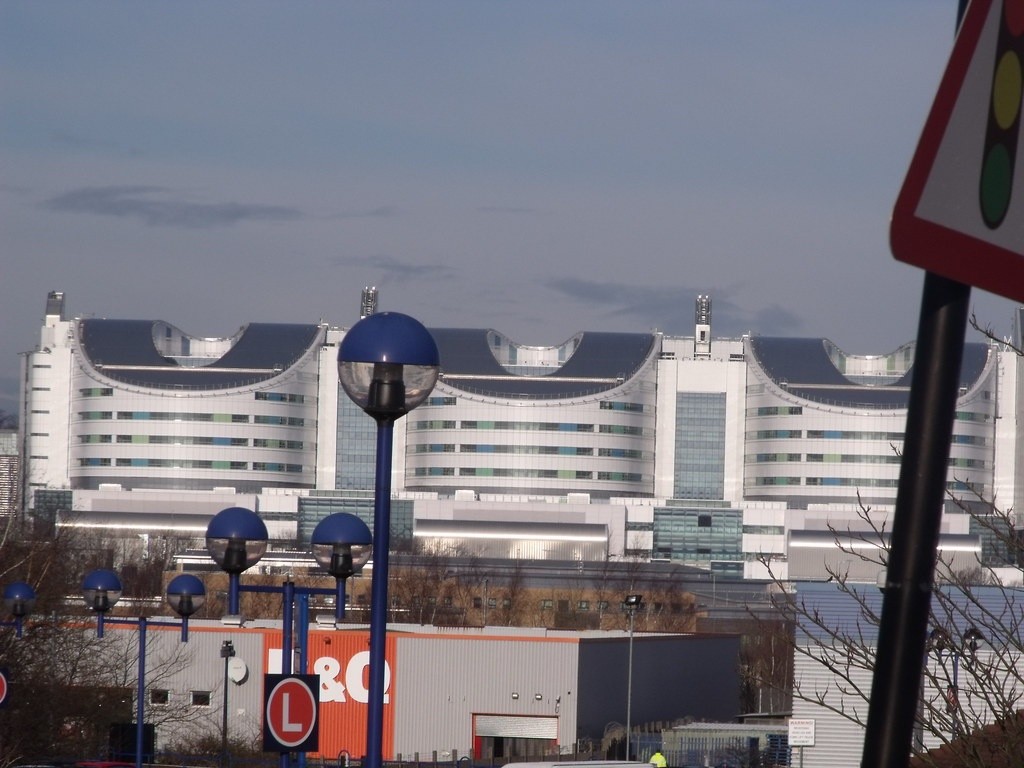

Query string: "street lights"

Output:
[206,508,268,616]
[338,311,441,768]
[620,593,643,762]
[82,570,123,638]
[166,574,206,642]
[4,582,36,639]
[311,513,372,622]
